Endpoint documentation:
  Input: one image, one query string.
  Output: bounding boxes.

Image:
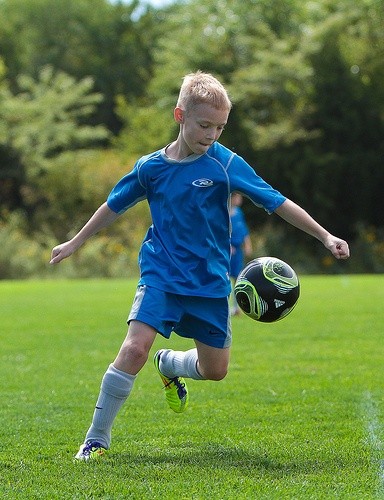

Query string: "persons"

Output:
[49,69,350,463]
[228,192,253,314]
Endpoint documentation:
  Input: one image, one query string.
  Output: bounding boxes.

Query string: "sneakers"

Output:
[73,443,108,462]
[154,349,189,413]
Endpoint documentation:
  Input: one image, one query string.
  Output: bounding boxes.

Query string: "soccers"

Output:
[234,256,300,323]
